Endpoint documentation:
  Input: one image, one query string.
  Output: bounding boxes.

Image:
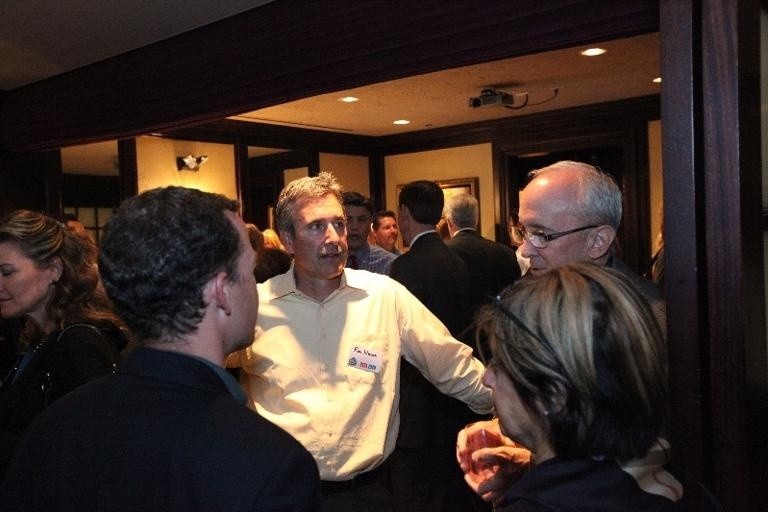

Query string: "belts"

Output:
[466,424,504,512]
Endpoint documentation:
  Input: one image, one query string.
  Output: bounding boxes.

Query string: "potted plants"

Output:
[396,177,480,253]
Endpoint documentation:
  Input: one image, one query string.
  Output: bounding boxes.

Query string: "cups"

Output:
[486,275,565,369]
[510,224,599,248]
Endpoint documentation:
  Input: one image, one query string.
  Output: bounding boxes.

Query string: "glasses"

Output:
[176,154,208,171]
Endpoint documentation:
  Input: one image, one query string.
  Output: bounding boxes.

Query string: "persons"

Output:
[261,229,287,255]
[509,208,532,278]
[651,207,666,284]
[455,158,667,366]
[435,211,450,242]
[226,171,493,512]
[455,260,723,512]
[442,187,522,321]
[370,210,404,256]
[0,185,323,512]
[245,224,291,284]
[0,208,130,467]
[61,221,130,339]
[387,181,485,512]
